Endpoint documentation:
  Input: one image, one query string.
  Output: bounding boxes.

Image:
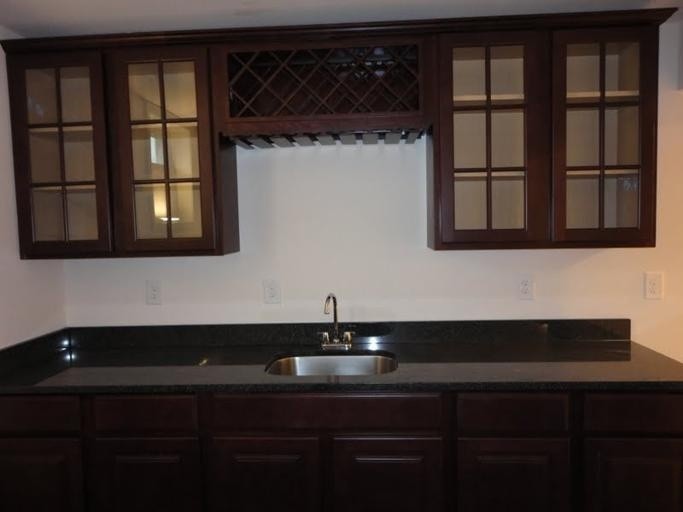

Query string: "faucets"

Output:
[324,293,340,344]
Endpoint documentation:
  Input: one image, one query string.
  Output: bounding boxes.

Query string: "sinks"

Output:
[265,350,399,375]
